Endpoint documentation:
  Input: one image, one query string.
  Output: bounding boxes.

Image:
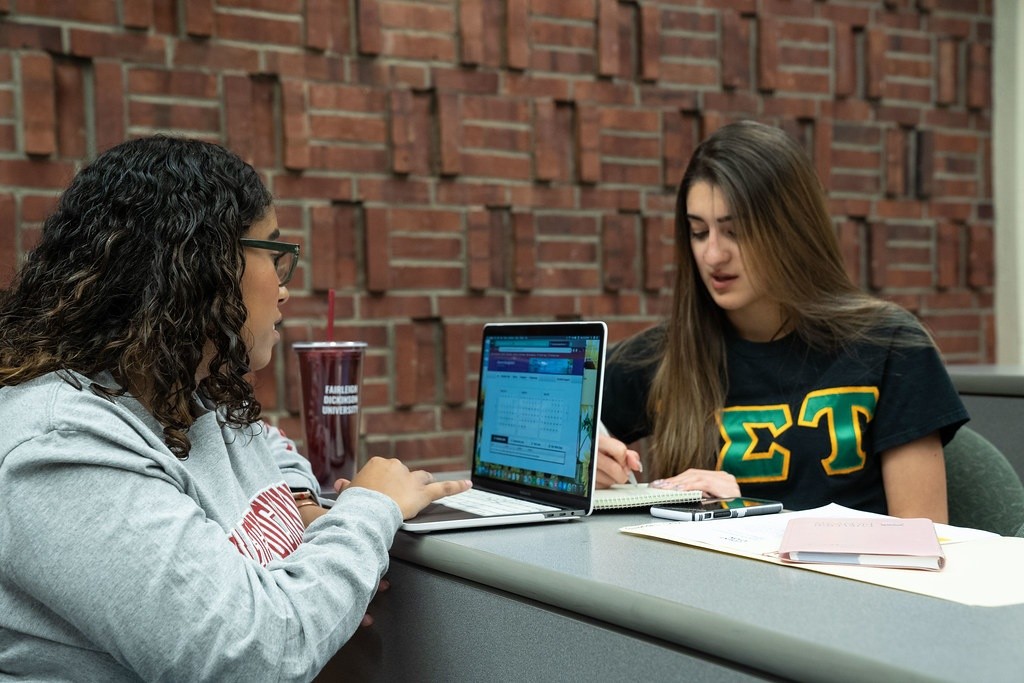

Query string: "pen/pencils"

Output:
[599,419,638,488]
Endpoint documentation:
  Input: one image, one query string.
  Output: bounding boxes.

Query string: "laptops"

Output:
[317,322,609,534]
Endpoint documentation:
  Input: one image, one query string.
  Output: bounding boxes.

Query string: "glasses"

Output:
[241,237,300,287]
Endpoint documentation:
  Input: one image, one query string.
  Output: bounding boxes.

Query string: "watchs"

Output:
[288,487,310,498]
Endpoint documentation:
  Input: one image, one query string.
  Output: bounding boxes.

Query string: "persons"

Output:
[594,118,972,524]
[0,134,473,683]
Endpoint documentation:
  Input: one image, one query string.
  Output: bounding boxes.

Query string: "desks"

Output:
[323,471,1024,683]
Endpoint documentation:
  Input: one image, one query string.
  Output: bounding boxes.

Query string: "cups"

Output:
[293,342,369,494]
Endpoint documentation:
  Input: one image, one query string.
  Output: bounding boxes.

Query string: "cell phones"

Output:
[650,497,784,522]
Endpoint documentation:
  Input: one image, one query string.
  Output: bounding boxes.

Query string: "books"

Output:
[592,483,707,510]
[778,518,945,571]
